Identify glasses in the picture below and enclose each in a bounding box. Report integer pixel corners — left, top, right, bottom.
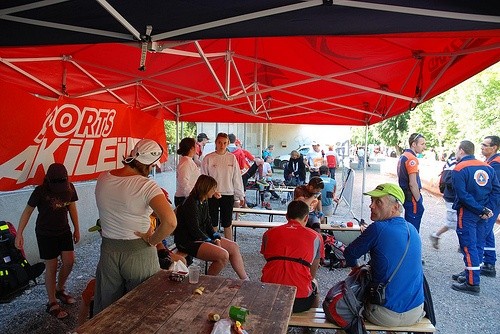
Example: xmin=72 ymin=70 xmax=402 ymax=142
xmin=481 ymin=142 xmax=490 ymax=147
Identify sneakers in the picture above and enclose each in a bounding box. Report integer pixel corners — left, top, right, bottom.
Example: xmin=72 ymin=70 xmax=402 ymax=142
xmin=256 ymin=179 xmax=269 ymax=186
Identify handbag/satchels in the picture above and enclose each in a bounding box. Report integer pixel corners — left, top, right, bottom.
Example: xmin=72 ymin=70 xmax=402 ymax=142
xmin=368 ymin=282 xmax=387 ymax=306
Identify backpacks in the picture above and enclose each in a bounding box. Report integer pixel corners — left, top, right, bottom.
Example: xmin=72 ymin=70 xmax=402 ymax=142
xmin=0 ymin=221 xmax=32 ymax=300
xmin=323 ymin=264 xmax=372 ymax=332
xmin=329 ymin=240 xmax=348 ymax=267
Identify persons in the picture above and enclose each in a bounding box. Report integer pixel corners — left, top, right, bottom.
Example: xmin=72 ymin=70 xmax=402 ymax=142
xmin=259 ymin=200 xmax=319 ymax=312
xmin=174 ymin=137 xmax=202 ymax=206
xmin=397 ymin=133 xmax=426 ymax=235
xmin=15 ymin=163 xmax=80 ymax=319
xmin=430 ymin=134 xmax=500 ymax=294
xmin=342 ymin=183 xmax=425 ymax=327
xmin=196 ymin=132 xmax=384 ymax=234
xmin=94 ymin=139 xmax=177 ymax=319
xmin=173 ymin=174 xmax=251 ymax=281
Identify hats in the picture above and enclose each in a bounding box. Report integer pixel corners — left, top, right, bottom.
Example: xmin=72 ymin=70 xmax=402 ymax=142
xmin=198 ymin=133 xmax=210 ymax=140
xmin=362 ymin=183 xmax=405 ymax=204
xmin=312 ymin=139 xmax=320 ymax=145
xmin=132 ymin=138 xmax=161 ymax=170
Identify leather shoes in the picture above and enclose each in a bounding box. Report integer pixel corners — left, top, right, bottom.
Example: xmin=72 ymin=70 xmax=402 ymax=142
xmin=451 ymin=281 xmax=480 ymax=296
xmin=458 ymin=276 xmax=466 ymax=283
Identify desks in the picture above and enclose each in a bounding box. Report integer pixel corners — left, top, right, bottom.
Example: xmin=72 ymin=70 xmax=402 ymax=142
xmin=72 ymin=268 xmax=297 ymax=334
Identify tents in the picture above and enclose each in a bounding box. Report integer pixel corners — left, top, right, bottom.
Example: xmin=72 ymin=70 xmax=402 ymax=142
xmin=0 ymin=0 xmax=500 ymax=236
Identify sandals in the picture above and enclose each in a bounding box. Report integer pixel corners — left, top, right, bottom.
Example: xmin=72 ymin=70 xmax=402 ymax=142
xmin=55 ymin=288 xmax=77 ymax=304
xmin=46 ymin=302 xmax=68 ymax=319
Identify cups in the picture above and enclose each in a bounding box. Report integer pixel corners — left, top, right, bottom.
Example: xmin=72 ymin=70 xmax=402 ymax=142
xmin=188 ymin=266 xmax=200 ymax=284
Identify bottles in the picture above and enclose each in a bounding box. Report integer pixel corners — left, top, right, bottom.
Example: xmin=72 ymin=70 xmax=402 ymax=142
xmin=208 ymin=313 xmax=220 ymax=321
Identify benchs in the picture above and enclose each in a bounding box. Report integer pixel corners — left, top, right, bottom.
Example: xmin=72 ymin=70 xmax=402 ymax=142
xmin=245 ymin=186 xmax=259 ymax=206
xmin=167 ymin=242 xmax=209 ymax=276
xmin=273 ymin=185 xmax=297 ymax=189
xmin=217 ymin=207 xmax=287 ymax=236
xmin=231 ymin=220 xmax=368 ymax=261
xmin=259 ymin=187 xmax=295 ymax=205
xmin=288 ymin=307 xmax=436 ymax=334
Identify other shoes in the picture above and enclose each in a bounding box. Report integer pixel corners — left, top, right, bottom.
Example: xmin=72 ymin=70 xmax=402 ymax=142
xmin=270 ymin=196 xmax=277 ymax=200
xmin=480 ymin=264 xmax=496 ymax=277
xmin=266 ymin=202 xmax=272 ymax=210
xmin=429 ymin=232 xmax=440 ymax=250
xmin=281 ymin=199 xmax=288 ymax=204
xmin=452 ymin=270 xmax=468 ymax=280
